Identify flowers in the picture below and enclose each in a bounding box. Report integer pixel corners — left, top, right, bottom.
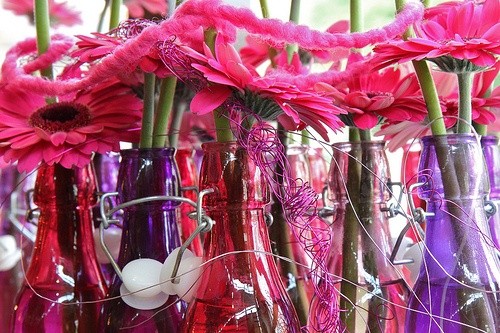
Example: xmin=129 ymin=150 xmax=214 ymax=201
xmin=0 ymin=0 xmax=500 ymax=333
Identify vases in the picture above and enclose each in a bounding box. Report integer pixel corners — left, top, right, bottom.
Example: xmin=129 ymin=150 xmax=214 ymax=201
xmin=190 ymin=135 xmax=303 ymax=333
xmin=178 ymin=148 xmax=204 ymax=255
xmin=97 ymin=144 xmax=195 ymax=333
xmin=306 ymin=139 xmax=409 ymax=333
xmin=405 ymin=130 xmax=500 ymax=333
xmin=8 ymin=152 xmax=110 ymax=333
xmin=259 ymin=140 xmax=322 ymax=321
xmin=484 ymin=132 xmax=500 ymax=248
